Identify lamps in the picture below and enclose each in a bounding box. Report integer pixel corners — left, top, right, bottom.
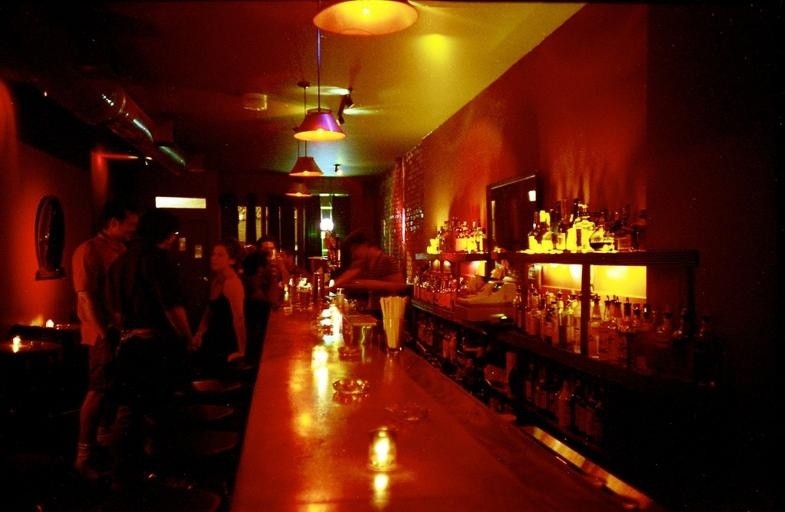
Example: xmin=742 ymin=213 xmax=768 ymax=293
xmin=283 ymin=0 xmax=421 ymax=200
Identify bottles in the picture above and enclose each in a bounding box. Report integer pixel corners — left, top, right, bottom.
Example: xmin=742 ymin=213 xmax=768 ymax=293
xmin=438 ymin=199 xmax=649 ymax=254
xmin=413 ymin=271 xmax=716 ymax=444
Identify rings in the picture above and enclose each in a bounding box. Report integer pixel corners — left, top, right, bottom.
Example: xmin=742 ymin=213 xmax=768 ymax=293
xmin=356 ymin=281 xmax=358 ymax=283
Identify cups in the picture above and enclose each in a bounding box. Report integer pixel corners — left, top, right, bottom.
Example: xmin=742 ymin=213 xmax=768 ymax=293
xmin=381 ymin=317 xmax=403 ymax=348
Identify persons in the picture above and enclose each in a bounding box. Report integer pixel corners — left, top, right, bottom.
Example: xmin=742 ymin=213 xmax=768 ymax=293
xmin=324 ymin=232 xmax=407 ymax=320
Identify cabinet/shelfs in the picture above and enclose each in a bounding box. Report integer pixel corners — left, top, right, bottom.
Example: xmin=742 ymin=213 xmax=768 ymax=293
xmin=410 ymin=248 xmax=700 ymax=461
